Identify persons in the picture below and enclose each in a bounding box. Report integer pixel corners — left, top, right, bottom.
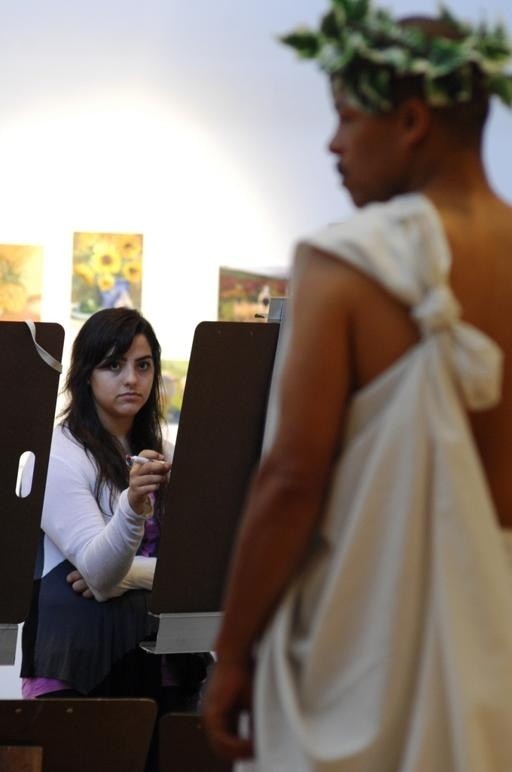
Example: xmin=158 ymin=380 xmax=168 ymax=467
xmin=199 ymin=1 xmax=511 ymax=772
xmin=13 ymin=308 xmax=220 ymax=714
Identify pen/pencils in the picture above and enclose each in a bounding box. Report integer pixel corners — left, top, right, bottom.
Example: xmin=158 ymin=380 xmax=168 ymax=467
xmin=127 ymin=456 xmax=167 ymax=464
